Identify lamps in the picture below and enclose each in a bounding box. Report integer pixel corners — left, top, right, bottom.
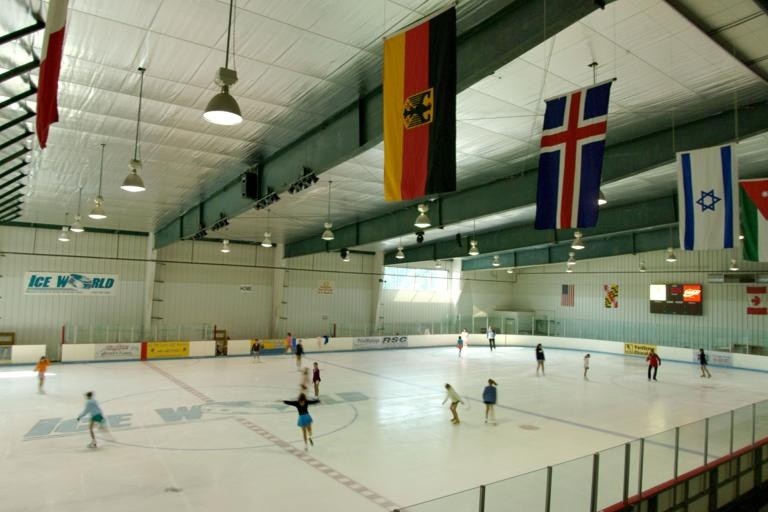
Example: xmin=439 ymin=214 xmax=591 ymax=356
xmin=219 ymin=239 xmax=231 ymax=254
xmin=57 ymin=212 xmax=72 ymax=242
xmin=87 ymin=143 xmax=109 ymax=221
xmin=339 ymin=225 xmax=746 ymax=277
xmin=118 ymin=66 xmax=147 ymax=194
xmin=321 ymin=180 xmax=334 ymax=241
xmin=260 ymin=208 xmax=273 ymax=248
xmin=198 ymin=1 xmax=245 ymax=127
xmin=70 ymin=187 xmax=85 ymax=233
xmin=413 ymin=201 xmax=433 ymax=230
xmin=586 ymin=59 xmax=608 ymax=208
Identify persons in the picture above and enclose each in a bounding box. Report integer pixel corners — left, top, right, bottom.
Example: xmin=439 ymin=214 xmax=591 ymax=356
xmin=482 ymin=378 xmax=498 ymax=424
xmin=535 ymin=344 xmax=546 ymax=376
xmin=487 ymin=326 xmax=496 ymax=350
xmin=33 ymin=356 xmax=50 ymax=391
xmin=583 ymin=353 xmax=591 ymax=380
xmin=697 ymin=347 xmax=713 ymax=378
xmin=279 ymin=332 xmax=321 ymax=450
xmin=77 ymin=392 xmax=108 ymax=449
xmin=646 ymin=348 xmax=661 ymax=382
xmin=252 ymin=339 xmax=262 ymax=361
xmin=441 ymin=383 xmax=465 ymax=426
xmin=455 ymin=335 xmax=464 ymax=359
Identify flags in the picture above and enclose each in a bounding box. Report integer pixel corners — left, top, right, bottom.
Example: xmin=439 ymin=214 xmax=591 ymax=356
xmin=561 ymin=284 xmax=575 ymax=307
xmin=532 ymin=76 xmax=617 ymax=231
xmin=382 ymin=7 xmax=458 ymax=203
xmin=738 ymin=177 xmax=768 ymax=263
xmin=675 ymin=143 xmax=741 ymax=251
xmin=746 ymin=286 xmax=768 ymax=315
xmin=35 ymin=0 xmax=69 ymax=148
